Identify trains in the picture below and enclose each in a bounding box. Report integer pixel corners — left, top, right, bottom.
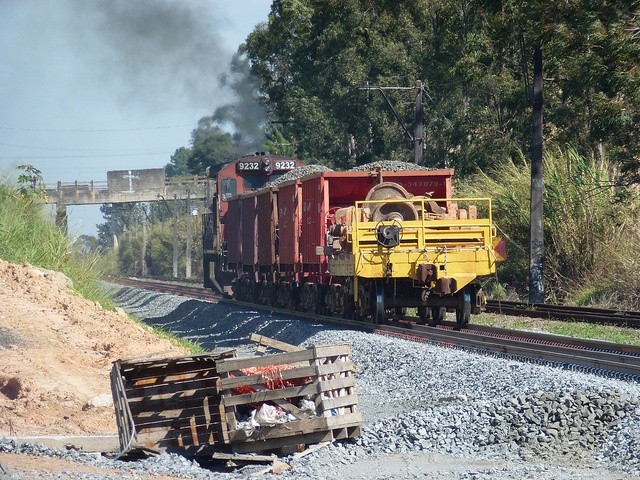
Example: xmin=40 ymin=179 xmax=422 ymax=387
xmin=202 ymin=155 xmax=497 ymax=325
